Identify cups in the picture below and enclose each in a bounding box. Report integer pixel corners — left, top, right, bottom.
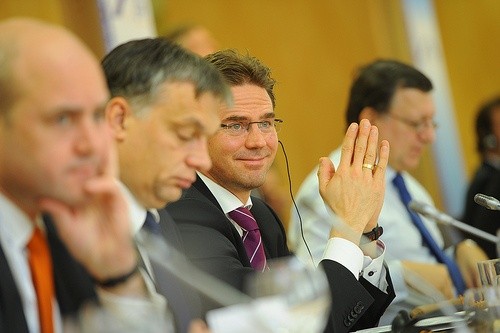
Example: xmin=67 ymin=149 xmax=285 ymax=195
xmin=475 ymin=256 xmax=500 ymax=333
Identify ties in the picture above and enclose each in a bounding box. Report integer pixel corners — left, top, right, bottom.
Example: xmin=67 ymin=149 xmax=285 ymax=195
xmin=27 ymin=227 xmax=54 ymax=333
xmin=229 ymin=208 xmax=270 ymax=273
xmin=392 ymin=172 xmax=465 ymax=296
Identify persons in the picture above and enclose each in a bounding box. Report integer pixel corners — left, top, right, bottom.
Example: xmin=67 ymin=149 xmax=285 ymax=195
xmin=0 ymin=17 xmax=500 ymax=333
xmin=162 ymin=48 xmax=397 ymax=332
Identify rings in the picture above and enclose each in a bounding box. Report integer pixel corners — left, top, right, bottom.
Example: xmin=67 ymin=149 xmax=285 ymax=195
xmin=361 ymin=163 xmax=374 ymax=172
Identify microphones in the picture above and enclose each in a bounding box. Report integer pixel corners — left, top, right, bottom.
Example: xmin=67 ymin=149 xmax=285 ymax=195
xmin=409 ymin=200 xmax=500 ymax=245
xmin=474 ymin=193 xmax=500 ymax=210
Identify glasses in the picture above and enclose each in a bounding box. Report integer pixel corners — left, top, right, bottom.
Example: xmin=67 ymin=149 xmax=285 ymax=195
xmin=386 ymin=111 xmax=439 ymax=131
xmin=220 ymin=119 xmax=283 ymax=136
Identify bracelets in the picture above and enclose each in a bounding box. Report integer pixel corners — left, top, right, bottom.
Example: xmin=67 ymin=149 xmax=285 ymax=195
xmin=359 ymin=223 xmax=383 ymax=245
